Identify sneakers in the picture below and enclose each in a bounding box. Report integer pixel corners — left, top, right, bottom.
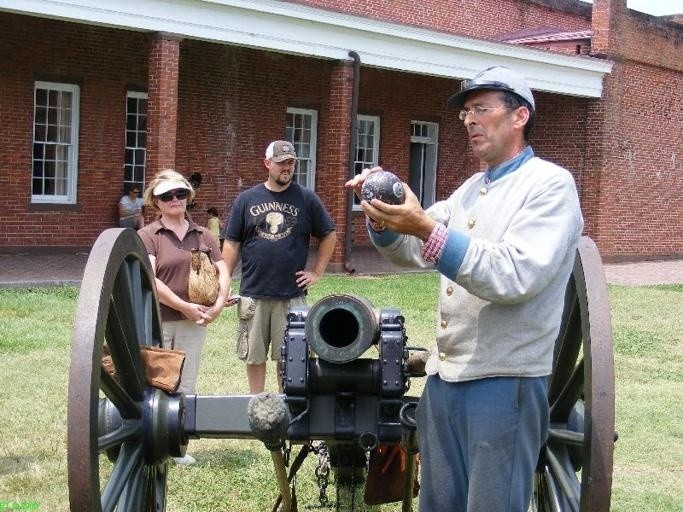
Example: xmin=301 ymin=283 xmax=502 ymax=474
xmin=173 ymin=453 xmax=196 ymax=465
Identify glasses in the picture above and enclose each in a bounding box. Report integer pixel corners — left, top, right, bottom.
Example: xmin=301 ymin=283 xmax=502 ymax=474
xmin=457 ymin=106 xmax=512 ymax=121
xmin=155 ymin=191 xmax=187 ymax=202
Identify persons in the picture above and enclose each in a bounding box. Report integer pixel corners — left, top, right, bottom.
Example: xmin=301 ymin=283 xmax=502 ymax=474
xmin=222 ymin=140 xmax=337 ymax=393
xmin=136 ymin=169 xmax=231 ymax=466
xmin=117 ymin=184 xmax=145 ymax=228
xmin=345 ymin=66 xmax=584 ymax=512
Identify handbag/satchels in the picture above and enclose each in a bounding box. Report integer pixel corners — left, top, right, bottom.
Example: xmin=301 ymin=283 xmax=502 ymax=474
xmin=186 ymin=247 xmax=220 ymax=307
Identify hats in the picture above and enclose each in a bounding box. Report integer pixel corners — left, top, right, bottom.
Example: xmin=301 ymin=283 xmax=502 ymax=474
xmin=265 ymin=140 xmax=299 ymax=165
xmin=151 ymin=179 xmax=192 ymax=196
xmin=446 ymin=66 xmax=536 ymax=114
